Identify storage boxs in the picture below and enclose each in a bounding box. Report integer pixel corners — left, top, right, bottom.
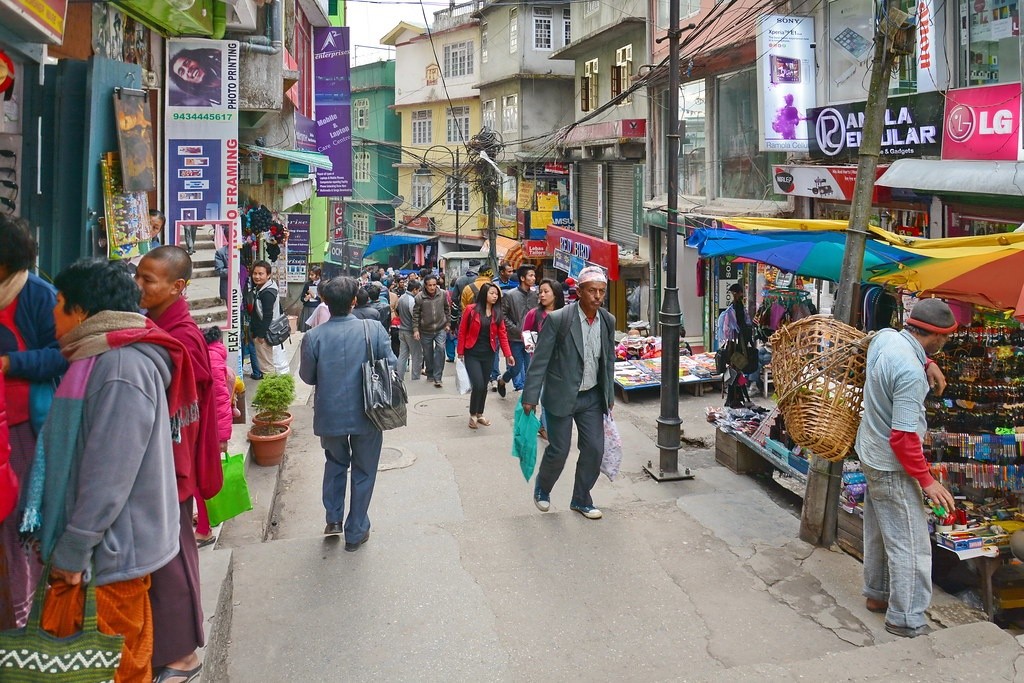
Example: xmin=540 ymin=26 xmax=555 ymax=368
xmin=715 ymin=428 xmax=776 ymax=476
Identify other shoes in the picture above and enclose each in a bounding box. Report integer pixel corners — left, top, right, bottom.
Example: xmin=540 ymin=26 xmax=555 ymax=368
xmin=885 ymin=619 xmax=931 ymax=638
xmin=492 ymin=381 xmax=497 ymax=392
xmin=865 ymin=598 xmax=888 ymax=613
xmin=423 ymin=359 xmax=453 ymax=386
xmin=250 ymin=372 xmax=260 ymax=379
xmin=345 ymin=531 xmax=369 ymax=552
xmin=324 ymin=522 xmax=343 ymax=536
xmin=498 ymin=379 xmax=506 ymax=398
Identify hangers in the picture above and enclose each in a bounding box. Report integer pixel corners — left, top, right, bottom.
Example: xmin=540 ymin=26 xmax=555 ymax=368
xmin=759 ymin=270 xmax=812 ymax=307
xmin=114 ymin=72 xmax=148 ymax=103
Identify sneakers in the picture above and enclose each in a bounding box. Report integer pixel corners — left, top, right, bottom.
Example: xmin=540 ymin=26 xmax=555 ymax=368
xmin=569 ymin=503 xmax=602 ymax=519
xmin=534 ymin=481 xmax=551 ymax=512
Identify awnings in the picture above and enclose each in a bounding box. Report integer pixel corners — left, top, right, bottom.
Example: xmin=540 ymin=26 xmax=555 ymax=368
xmin=239 ymin=143 xmax=332 ymax=172
xmin=874 ymin=159 xmax=1024 ymax=198
xmin=687 ymin=217 xmax=1024 ymax=322
xmin=480 ymin=235 xmax=523 ymax=270
xmin=363 ymin=230 xmax=438 ymax=266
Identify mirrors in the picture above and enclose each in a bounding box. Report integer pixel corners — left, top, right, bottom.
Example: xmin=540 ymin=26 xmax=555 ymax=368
xmin=175 ymin=219 xmax=234 ymax=332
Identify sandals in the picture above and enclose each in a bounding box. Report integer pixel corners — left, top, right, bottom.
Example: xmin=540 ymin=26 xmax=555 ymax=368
xmin=477 ymin=416 xmax=491 ymax=426
xmin=468 ymin=416 xmax=479 ymax=429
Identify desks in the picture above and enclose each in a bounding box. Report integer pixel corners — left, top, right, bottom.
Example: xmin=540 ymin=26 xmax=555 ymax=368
xmin=930 ymin=540 xmax=1024 ymax=622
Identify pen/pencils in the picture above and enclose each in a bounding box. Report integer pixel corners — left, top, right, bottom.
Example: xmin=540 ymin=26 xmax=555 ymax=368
xmin=924 ymin=432 xmax=1024 ymax=494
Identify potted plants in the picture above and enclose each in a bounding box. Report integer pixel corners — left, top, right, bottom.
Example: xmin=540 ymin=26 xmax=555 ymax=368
xmin=246 ymin=377 xmax=292 ymax=468
xmin=251 ymin=373 xmax=294 ymax=425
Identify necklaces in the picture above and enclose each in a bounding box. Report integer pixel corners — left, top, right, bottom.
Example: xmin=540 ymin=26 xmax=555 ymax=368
xmin=487 ymin=310 xmax=491 ymax=315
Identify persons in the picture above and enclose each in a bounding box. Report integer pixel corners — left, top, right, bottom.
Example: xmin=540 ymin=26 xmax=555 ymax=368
xmin=239 ymin=259 xmax=580 ymax=440
xmin=778 ymin=62 xmax=790 ymax=73
xmin=0 ymin=209 xmax=233 ymax=683
xmin=182 ymin=225 xmax=228 ymax=309
xmin=92 ymin=11 xmax=148 ymax=67
xmin=298 ymin=275 xmax=399 ymax=552
xmin=522 ymin=267 xmax=614 ymax=520
xmin=853 ymin=299 xmax=956 ymax=638
xmin=779 ymin=94 xmax=799 ymax=140
xmin=169 ymin=48 xmax=222 ymax=107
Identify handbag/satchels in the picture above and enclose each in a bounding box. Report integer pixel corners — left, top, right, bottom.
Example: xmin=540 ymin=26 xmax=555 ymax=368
xmin=715 ymin=330 xmax=759 ymax=388
xmin=523 ymin=330 xmax=538 ymax=353
xmin=599 ymin=408 xmax=621 ymax=483
xmin=204 ymin=450 xmax=253 ymax=528
xmin=455 ymin=356 xmax=474 ymax=396
xmin=363 ymin=318 xmax=408 ymax=432
xmin=0 ymin=557 xmax=124 ymax=683
xmin=265 ymin=313 xmax=293 ymax=350
xmin=511 ymin=393 xmax=541 ymax=483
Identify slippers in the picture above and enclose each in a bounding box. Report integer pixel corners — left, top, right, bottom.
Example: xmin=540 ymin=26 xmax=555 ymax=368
xmin=193 ymin=533 xmax=216 ymax=548
xmin=193 ymin=515 xmax=218 ymax=527
xmin=153 ymin=661 xmax=204 ymax=683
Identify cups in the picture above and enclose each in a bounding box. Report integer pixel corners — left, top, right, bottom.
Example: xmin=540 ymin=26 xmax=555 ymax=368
xmin=935 ymin=524 xmax=952 ymax=533
xmin=954 ymin=524 xmax=967 ymax=530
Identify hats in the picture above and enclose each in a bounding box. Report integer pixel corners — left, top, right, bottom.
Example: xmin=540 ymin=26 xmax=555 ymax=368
xmin=579 ymin=266 xmax=607 ymax=286
xmin=905 ymin=296 xmax=957 ymax=334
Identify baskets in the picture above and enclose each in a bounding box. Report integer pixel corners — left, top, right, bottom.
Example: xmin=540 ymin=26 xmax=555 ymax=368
xmin=765 ymin=313 xmax=874 ymax=464
